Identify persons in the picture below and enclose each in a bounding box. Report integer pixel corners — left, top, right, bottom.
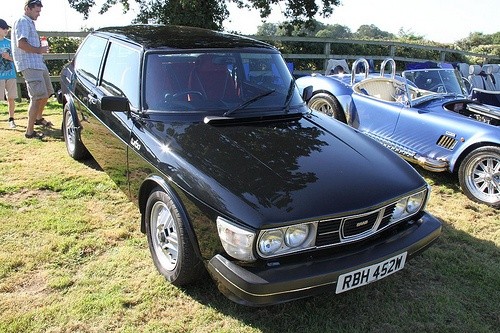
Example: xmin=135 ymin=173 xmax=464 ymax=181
xmin=11 ymin=0 xmax=54 ymax=139
xmin=0 ymin=18 xmax=17 ymax=128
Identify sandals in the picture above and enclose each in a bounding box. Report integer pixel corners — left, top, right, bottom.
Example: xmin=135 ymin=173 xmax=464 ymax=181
xmin=35 ymin=117 xmax=53 ymax=127
xmin=24 ymin=130 xmax=45 ymax=139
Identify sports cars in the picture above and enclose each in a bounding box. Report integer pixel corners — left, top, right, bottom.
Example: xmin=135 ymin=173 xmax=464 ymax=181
xmin=292 ymin=57 xmax=500 ymax=207
xmin=407 ymin=60 xmax=500 ymax=109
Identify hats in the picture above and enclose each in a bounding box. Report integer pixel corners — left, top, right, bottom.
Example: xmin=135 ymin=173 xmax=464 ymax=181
xmin=0 ymin=19 xmax=12 ymax=29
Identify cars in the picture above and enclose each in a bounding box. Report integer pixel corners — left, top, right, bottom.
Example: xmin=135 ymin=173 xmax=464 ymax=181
xmin=56 ymin=25 xmax=444 ymax=308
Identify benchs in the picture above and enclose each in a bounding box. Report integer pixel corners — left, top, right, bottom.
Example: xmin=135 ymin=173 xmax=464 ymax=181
xmin=352 ymin=77 xmax=420 ymax=100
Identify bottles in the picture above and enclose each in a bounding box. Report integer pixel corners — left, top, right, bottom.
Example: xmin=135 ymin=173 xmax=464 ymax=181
xmin=41 ymin=38 xmax=48 ymax=55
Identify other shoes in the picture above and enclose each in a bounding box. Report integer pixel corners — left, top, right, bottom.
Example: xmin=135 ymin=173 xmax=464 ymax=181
xmin=8 ymin=118 xmax=16 ymax=129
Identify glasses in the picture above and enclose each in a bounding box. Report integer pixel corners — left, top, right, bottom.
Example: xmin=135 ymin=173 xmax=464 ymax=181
xmin=4 ymin=28 xmax=10 ymax=30
xmin=30 ymin=0 xmax=41 ymax=5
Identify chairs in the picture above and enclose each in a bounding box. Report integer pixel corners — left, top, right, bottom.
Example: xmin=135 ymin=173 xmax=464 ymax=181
xmin=122 ymin=53 xmax=177 ymax=109
xmin=188 ymin=54 xmax=237 ymax=101
xmin=468 ymin=65 xmax=496 ymax=91
